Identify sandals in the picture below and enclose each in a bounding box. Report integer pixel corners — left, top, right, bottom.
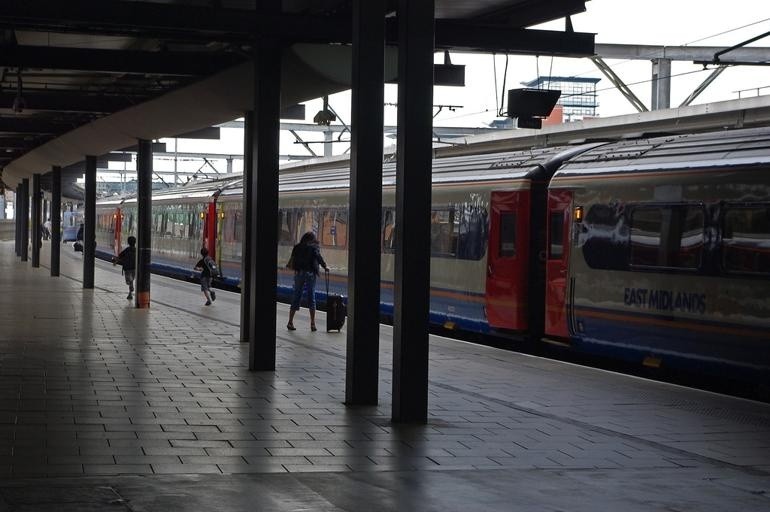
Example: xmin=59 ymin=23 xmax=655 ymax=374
xmin=286 ymin=322 xmax=297 ymax=330
xmin=309 ymin=322 xmax=317 ymax=332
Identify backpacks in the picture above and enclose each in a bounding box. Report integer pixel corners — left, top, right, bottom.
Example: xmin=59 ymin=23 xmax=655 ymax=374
xmin=203 ymin=255 xmax=219 ymax=279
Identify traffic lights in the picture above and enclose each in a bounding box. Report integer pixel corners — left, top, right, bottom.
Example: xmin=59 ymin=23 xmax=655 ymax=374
xmin=507 ymin=84 xmax=563 ymax=122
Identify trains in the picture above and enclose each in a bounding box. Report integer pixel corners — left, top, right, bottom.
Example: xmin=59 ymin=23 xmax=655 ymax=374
xmin=95 ymin=126 xmax=770 ymax=395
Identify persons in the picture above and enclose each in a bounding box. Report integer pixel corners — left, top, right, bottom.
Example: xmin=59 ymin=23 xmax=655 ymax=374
xmin=118 ymin=236 xmax=136 ymax=299
xmin=286 ymin=231 xmax=330 ymax=331
xmin=194 ymin=247 xmax=217 ymax=306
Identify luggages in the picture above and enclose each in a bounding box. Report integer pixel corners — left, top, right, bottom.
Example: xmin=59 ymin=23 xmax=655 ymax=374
xmin=325 ymin=291 xmax=347 ymax=334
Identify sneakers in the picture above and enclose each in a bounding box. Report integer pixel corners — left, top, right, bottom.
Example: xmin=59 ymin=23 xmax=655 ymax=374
xmin=129 ymin=287 xmax=134 ymax=292
xmin=210 ymin=291 xmax=216 ymax=301
xmin=126 ymin=295 xmax=133 ymax=299
xmin=205 ymin=300 xmax=211 ymax=306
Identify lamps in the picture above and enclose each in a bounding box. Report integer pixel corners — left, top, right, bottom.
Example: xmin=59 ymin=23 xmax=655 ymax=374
xmin=13 ymin=77 xmax=24 ymax=112
xmin=314 ymin=96 xmax=335 ymax=126
xmin=493 ymin=53 xmax=561 ymax=129
